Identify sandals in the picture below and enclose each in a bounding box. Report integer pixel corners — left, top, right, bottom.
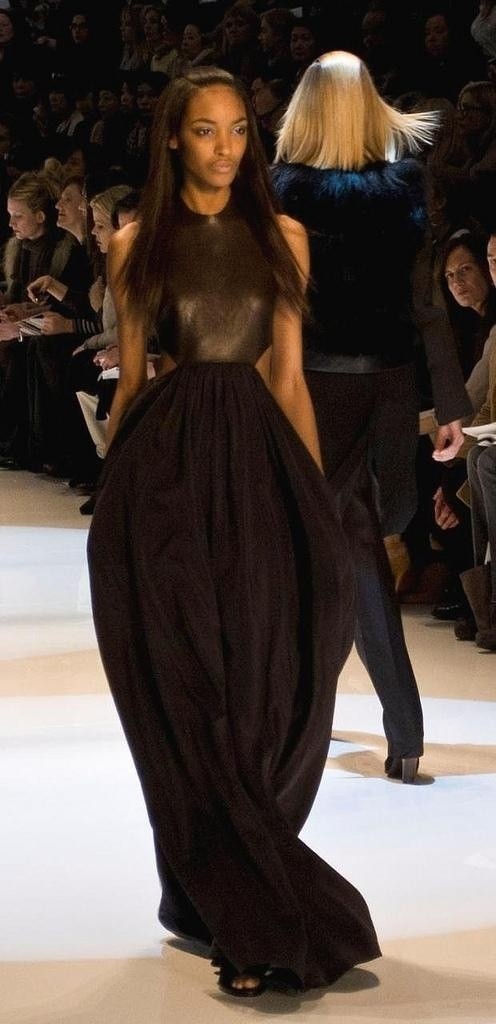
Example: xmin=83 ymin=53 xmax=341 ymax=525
xmin=218 ymin=954 xmax=268 ymax=997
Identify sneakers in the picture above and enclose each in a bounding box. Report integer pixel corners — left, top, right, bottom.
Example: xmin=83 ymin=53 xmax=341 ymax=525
xmin=476 ymin=624 xmax=495 ymax=647
xmin=453 ymin=613 xmax=476 ymax=640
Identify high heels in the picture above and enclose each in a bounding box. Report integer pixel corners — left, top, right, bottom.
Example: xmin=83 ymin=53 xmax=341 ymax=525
xmin=384 ymin=756 xmax=419 ymax=783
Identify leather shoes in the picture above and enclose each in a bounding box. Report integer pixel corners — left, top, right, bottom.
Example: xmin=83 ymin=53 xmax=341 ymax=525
xmin=430 ymin=601 xmax=464 ymax=619
xmin=79 ymin=495 xmax=96 ymax=514
